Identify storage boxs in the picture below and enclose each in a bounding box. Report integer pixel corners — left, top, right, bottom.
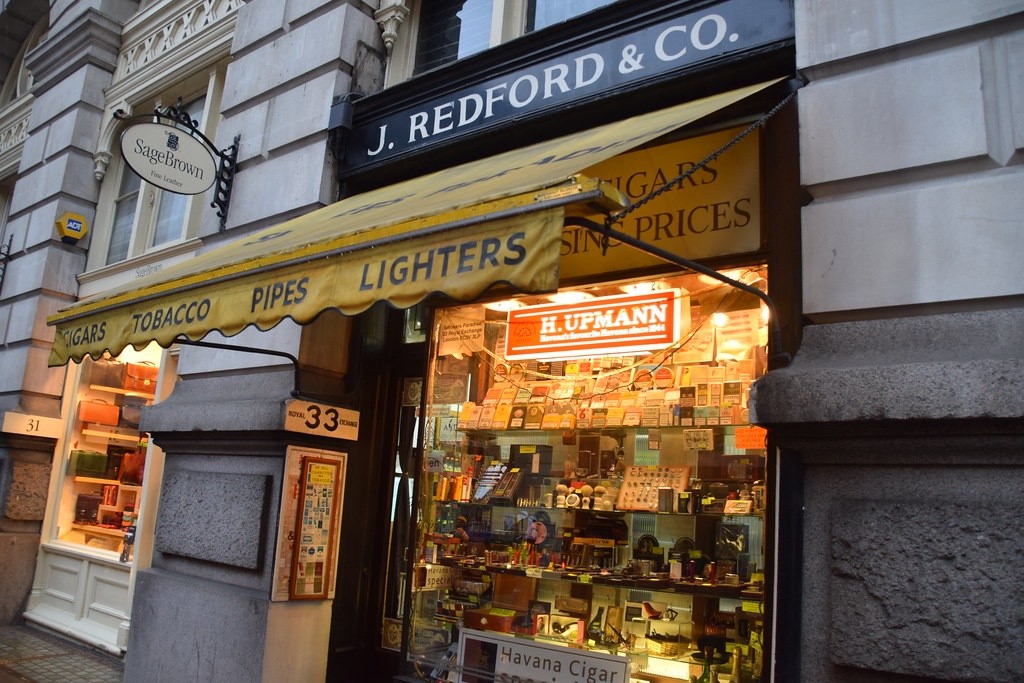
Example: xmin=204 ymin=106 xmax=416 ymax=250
xmin=463 ymin=573 xmax=540 ymax=633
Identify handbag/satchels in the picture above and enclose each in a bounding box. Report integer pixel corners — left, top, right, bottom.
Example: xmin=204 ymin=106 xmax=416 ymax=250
xmin=78 ymin=398 xmax=120 ymax=427
xmin=107 ymin=450 xmax=128 ymax=480
xmin=82 ymin=351 xmax=125 ymax=390
xmin=122 ymin=360 xmax=159 ymax=395
xmin=74 ymin=491 xmax=104 ymax=526
xmin=117 ymin=432 xmax=149 ymax=486
xmin=65 ymin=449 xmax=109 ymax=479
xmin=119 ymin=403 xmax=142 ymax=430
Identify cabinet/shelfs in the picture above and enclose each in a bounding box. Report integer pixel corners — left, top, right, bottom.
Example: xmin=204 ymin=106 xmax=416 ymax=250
xmin=425 ymin=422 xmax=772 ymax=683
xmin=70 ymin=384 xmax=156 ymax=542
